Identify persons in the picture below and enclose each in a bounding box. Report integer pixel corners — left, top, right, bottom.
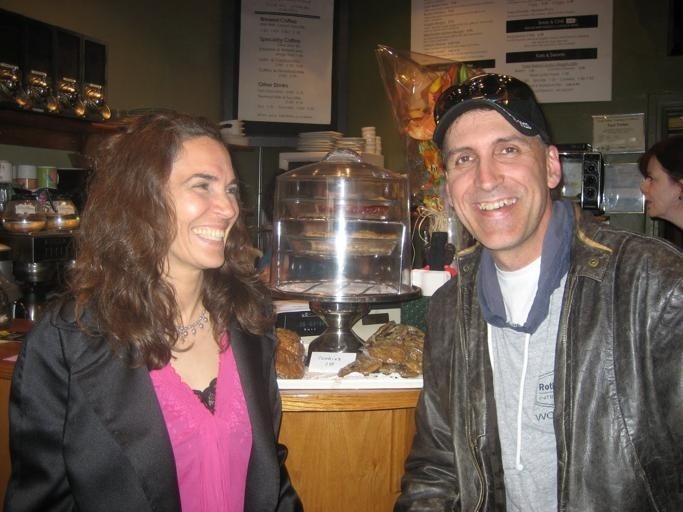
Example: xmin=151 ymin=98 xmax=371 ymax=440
xmin=393 ymin=73 xmax=683 ymax=512
xmin=638 ymin=136 xmax=683 ymax=231
xmin=4 ymin=109 xmax=304 ymax=512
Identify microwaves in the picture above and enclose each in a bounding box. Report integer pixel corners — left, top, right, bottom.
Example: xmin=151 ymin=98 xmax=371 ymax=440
xmin=553 ymin=143 xmax=604 ymax=219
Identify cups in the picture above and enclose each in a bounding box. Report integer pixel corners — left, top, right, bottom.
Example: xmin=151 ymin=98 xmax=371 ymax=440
xmin=362 ymin=127 xmax=382 ymax=154
xmin=0 ymin=160 xmax=60 ymax=210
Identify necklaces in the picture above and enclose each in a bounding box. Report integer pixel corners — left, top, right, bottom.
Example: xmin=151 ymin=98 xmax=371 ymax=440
xmin=175 ymin=309 xmax=208 ymax=336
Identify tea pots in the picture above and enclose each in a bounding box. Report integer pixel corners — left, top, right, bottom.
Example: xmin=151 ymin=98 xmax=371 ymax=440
xmin=0 ymin=186 xmax=81 ymax=234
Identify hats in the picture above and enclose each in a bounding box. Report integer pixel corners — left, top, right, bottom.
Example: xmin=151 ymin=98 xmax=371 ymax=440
xmin=432 ymin=77 xmax=551 ymax=148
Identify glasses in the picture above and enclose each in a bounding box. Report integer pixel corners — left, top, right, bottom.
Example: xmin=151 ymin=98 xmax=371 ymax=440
xmin=434 ymin=74 xmax=549 ymax=125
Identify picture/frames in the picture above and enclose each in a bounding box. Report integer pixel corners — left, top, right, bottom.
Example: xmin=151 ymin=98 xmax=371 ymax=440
xmin=232 ymin=1 xmax=339 ymax=132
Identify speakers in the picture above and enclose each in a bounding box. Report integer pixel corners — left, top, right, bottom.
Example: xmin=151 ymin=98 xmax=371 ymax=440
xmin=581 ymin=153 xmax=603 ymax=215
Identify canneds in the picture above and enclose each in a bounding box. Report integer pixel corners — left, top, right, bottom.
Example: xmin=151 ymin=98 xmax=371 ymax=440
xmin=1 ymin=194 xmax=81 ymax=233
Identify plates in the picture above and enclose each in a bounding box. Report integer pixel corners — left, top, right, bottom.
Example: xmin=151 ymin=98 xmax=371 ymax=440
xmin=218 ymin=119 xmax=247 ymax=139
xmin=294 ymin=131 xmax=366 ymax=155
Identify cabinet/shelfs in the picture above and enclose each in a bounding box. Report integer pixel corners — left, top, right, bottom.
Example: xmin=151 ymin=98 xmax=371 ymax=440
xmin=268 ymin=150 xmax=422 ymax=365
